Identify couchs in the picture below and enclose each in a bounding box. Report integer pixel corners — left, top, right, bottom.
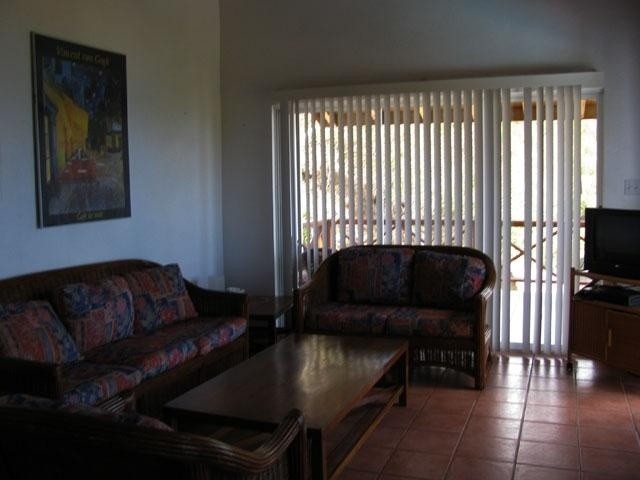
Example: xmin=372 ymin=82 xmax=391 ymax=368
xmin=2 ymin=257 xmax=250 ymax=414
xmin=1 ymin=390 xmax=310 ymax=480
xmin=294 ymin=243 xmax=494 ymax=390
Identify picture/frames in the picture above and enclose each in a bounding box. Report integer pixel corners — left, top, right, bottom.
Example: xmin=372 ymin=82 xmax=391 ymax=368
xmin=31 ymin=30 xmax=131 ymax=227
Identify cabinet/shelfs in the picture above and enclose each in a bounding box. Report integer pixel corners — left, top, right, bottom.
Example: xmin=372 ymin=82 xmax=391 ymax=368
xmin=567 ymin=270 xmax=639 ymax=375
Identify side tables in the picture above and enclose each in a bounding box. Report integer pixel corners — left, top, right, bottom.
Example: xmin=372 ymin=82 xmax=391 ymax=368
xmin=239 ymin=294 xmax=298 ymax=349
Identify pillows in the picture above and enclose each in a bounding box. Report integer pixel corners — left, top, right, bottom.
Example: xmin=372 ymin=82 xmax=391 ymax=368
xmin=5 ymin=263 xmax=198 ymax=374
xmin=335 ymin=247 xmax=481 ymax=310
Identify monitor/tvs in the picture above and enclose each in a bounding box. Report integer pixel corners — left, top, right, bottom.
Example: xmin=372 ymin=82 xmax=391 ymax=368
xmin=583 ymin=207 xmax=640 ymax=281
